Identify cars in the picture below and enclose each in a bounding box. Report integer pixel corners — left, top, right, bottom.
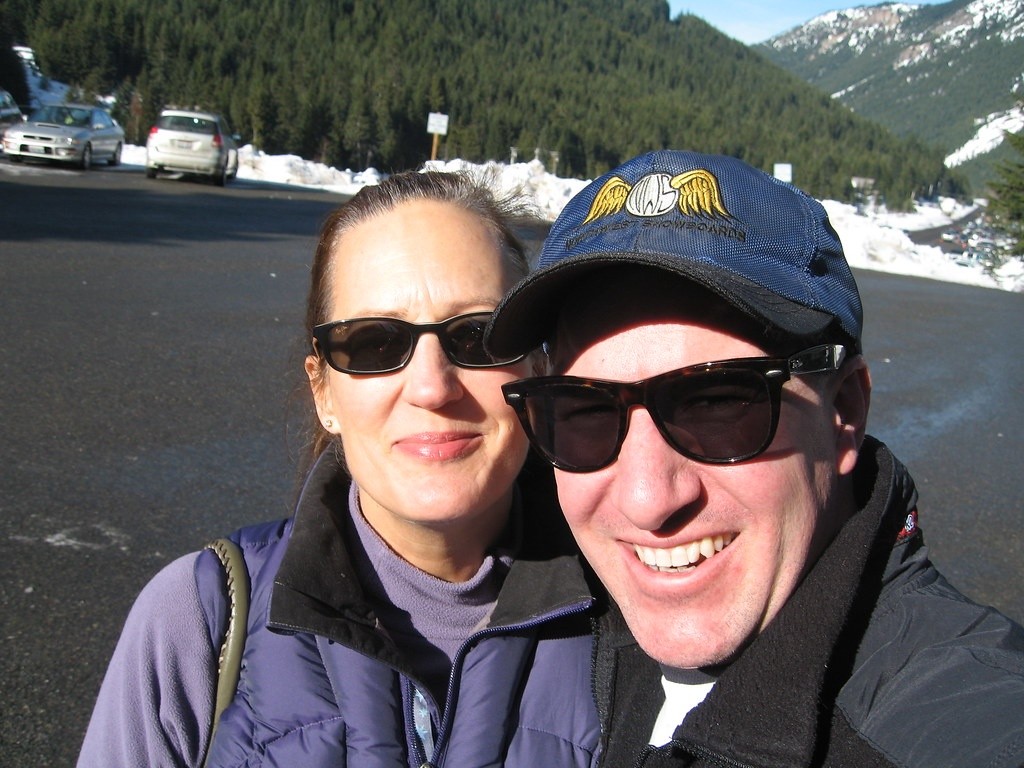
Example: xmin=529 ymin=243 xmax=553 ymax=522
xmin=143 ymin=110 xmax=242 ymax=187
xmin=0 ymin=89 xmax=125 ymax=171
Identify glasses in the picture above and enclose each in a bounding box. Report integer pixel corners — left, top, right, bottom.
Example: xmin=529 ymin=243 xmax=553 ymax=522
xmin=501 ymin=344 xmax=850 ymax=474
xmin=312 ymin=311 xmax=533 ymax=376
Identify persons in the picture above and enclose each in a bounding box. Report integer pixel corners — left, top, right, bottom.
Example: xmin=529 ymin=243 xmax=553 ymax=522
xmin=481 ymin=148 xmax=1023 ymax=767
xmin=78 ymin=164 xmax=601 ymax=767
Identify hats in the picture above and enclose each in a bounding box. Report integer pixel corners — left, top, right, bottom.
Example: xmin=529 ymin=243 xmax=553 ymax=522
xmin=485 ymin=150 xmax=863 ymax=370
